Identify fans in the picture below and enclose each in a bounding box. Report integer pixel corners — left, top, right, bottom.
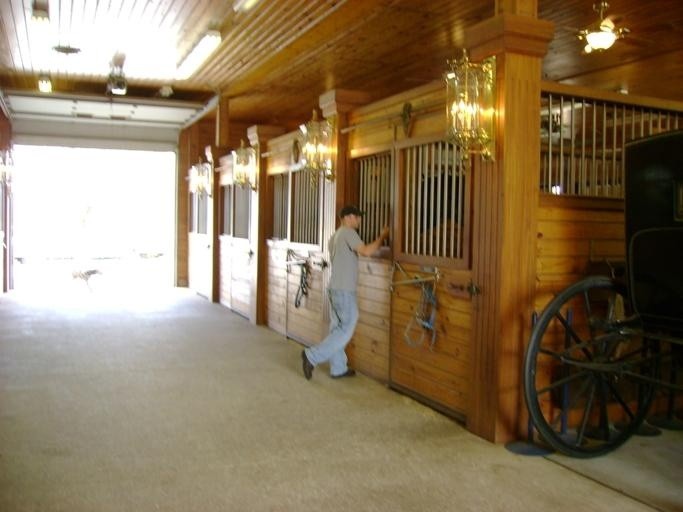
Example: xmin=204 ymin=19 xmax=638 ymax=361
xmin=564 ymin=2 xmax=654 ymax=51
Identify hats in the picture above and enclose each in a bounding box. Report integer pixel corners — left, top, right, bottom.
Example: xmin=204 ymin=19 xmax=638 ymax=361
xmin=340 ymin=206 xmax=364 ymax=218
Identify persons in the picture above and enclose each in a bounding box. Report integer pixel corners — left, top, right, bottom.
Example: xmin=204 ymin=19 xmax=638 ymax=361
xmin=300 ymin=202 xmax=388 ymax=381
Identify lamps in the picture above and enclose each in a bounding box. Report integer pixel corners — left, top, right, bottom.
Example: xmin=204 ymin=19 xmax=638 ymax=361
xmin=174 ymin=30 xmax=222 ymax=81
xmin=107 ymin=76 xmax=129 ymax=96
xmin=38 ymin=75 xmax=52 ymax=93
xmin=31 ymin=1 xmax=50 ymax=22
xmin=232 ymin=0 xmax=260 ymax=14
xmin=573 ymin=27 xmax=630 ymax=57
xmin=301 ymin=108 xmax=335 ymax=188
xmin=229 ymin=138 xmax=257 ymax=194
xmin=189 ymin=155 xmax=212 ymax=199
xmin=441 ymin=48 xmax=497 ymax=163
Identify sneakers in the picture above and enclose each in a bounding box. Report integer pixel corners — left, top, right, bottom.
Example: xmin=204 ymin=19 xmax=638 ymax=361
xmin=302 ymin=350 xmax=313 ymax=380
xmin=332 ymin=369 xmax=356 ymax=379
